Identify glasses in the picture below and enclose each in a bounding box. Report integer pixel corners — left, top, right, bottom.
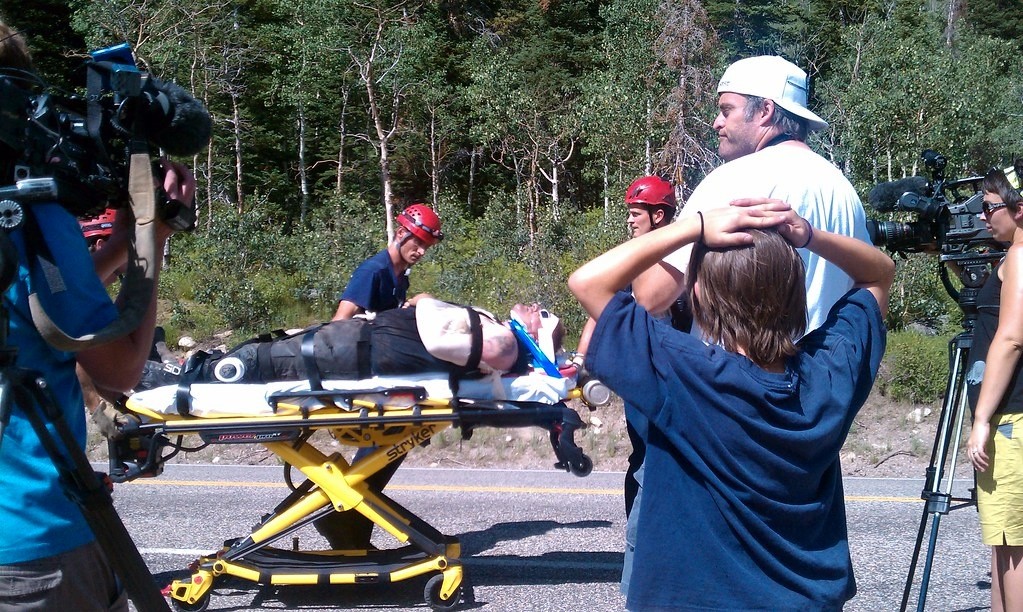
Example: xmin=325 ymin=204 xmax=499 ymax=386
xmin=539 ymin=309 xmax=549 ymax=319
xmin=982 ymin=201 xmax=1008 ymax=214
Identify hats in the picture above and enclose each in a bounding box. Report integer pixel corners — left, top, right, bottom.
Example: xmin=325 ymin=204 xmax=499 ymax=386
xmin=717 ymin=55 xmax=830 ymax=130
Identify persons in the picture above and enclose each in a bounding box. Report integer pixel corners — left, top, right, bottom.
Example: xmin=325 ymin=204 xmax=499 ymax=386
xmin=0 ymin=22 xmax=196 ymax=612
xmin=559 ymin=175 xmax=693 ymax=525
xmin=568 ymin=197 xmax=896 ymax=612
xmin=966 ymin=166 xmax=1023 ymax=612
xmin=312 ymin=204 xmax=445 ymax=551
xmin=618 ymin=54 xmax=875 ymax=599
xmin=132 ymin=292 xmax=565 ymax=393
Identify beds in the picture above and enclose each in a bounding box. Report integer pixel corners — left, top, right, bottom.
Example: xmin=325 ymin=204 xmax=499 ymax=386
xmin=108 ymin=374 xmax=610 ymax=612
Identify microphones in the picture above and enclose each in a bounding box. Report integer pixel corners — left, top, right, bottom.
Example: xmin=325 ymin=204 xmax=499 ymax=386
xmin=868 ymin=176 xmax=933 ymax=213
xmin=125 ymin=78 xmax=214 ymax=157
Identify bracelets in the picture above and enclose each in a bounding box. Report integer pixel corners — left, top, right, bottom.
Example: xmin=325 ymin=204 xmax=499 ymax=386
xmin=800 ymin=218 xmax=813 ymax=248
xmin=697 ymin=211 xmax=704 ymax=234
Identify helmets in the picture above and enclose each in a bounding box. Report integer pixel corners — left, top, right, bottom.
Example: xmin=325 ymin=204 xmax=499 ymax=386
xmin=78 ymin=208 xmax=118 ymax=239
xmin=397 ymin=204 xmax=444 ymax=245
xmin=625 ymin=177 xmax=676 ymax=208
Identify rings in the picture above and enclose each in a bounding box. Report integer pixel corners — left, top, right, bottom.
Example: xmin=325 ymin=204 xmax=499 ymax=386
xmin=972 ymin=448 xmax=978 ymax=454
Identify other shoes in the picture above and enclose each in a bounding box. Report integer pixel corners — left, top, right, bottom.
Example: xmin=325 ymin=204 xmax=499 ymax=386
xmin=312 ymin=510 xmax=381 ymax=557
xmin=148 ymin=326 xmax=181 ymax=368
